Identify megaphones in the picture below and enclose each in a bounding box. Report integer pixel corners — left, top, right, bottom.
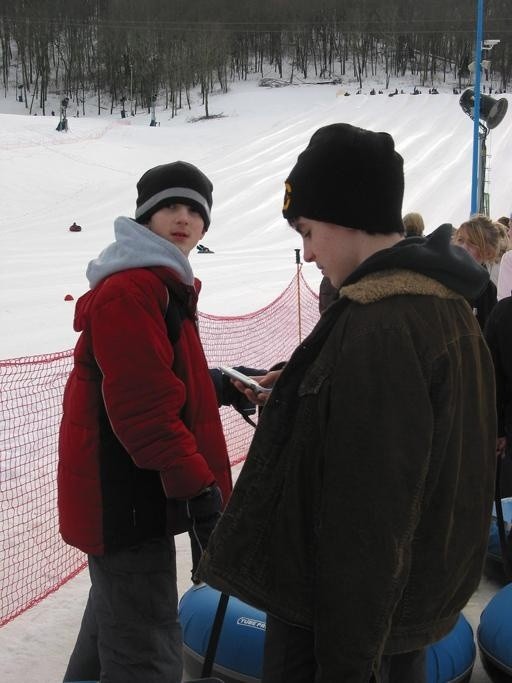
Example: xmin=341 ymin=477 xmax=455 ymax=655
xmin=459 ymin=89 xmax=508 ymax=129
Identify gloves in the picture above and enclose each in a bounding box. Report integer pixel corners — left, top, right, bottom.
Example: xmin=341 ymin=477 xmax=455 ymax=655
xmin=224 ymin=364 xmax=268 ymax=416
xmin=184 ymin=484 xmax=224 ymax=587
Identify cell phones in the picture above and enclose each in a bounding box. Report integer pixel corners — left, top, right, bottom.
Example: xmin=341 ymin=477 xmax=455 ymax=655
xmin=217 ymin=365 xmax=273 ymax=399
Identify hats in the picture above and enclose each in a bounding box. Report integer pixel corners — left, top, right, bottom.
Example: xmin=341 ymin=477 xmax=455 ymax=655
xmin=134 ymin=160 xmax=213 ymax=233
xmin=282 ymin=123 xmax=405 ymax=234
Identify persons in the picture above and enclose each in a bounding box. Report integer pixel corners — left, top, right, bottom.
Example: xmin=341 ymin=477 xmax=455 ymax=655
xmin=452 ymin=79 xmax=471 ymax=94
xmin=34 ymin=112 xmax=38 ymax=116
xmin=413 ymin=87 xmax=421 ymax=95
xmin=55 ymin=118 xmax=68 ymax=132
xmin=344 ymin=89 xmax=362 ymax=96
xmin=57 ymin=160 xmax=270 ymax=683
xmin=149 ymin=119 xmax=161 ymax=127
xmin=483 ymin=84 xmax=506 ymax=94
xmin=51 ymin=109 xmax=55 ymax=116
xmin=428 ymin=87 xmax=439 ymax=94
xmin=369 ymin=88 xmax=383 ymax=95
xmin=394 ymin=88 xmax=405 ymax=94
xmin=121 ymin=109 xmax=135 ymax=118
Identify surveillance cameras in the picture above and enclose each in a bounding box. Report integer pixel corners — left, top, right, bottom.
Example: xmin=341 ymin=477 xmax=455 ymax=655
xmin=484 ymin=39 xmax=500 ymax=46
